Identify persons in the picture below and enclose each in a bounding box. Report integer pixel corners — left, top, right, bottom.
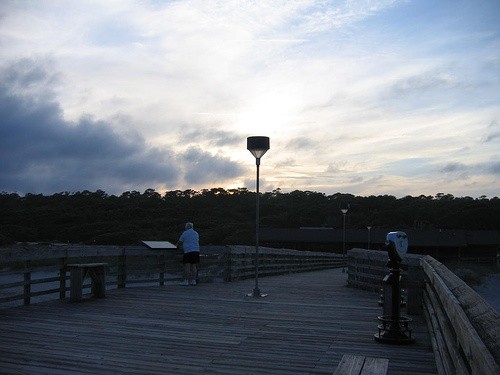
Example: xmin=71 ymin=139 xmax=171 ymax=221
xmin=177 ymin=223 xmax=199 ymax=285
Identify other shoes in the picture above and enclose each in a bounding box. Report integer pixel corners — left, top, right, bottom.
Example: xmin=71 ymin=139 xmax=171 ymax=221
xmin=179 ymin=280 xmax=196 ymax=286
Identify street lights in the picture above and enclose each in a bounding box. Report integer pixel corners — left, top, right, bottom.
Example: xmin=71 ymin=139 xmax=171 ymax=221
xmin=247 ymin=137 xmax=270 ymax=297
xmin=339 ymin=201 xmax=349 ymax=274
xmin=366 ymin=225 xmax=372 ymax=250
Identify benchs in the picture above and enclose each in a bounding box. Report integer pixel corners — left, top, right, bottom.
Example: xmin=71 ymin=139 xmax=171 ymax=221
xmin=66 ymin=262 xmax=108 ymax=302
